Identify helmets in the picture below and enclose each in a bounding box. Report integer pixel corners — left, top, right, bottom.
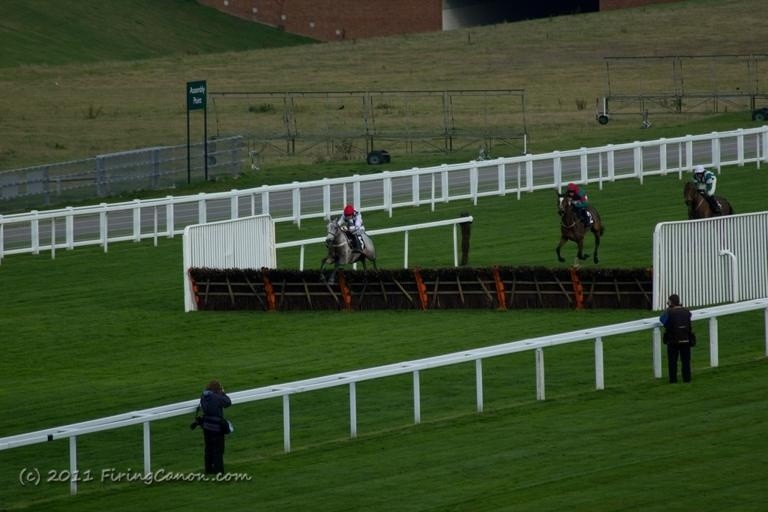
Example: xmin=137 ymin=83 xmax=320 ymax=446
xmin=694 ymin=165 xmax=705 ymax=174
xmin=344 ymin=205 xmax=355 ymax=217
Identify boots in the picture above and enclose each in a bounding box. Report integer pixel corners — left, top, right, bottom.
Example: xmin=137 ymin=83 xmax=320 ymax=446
xmin=353 ymin=239 xmax=363 ymax=253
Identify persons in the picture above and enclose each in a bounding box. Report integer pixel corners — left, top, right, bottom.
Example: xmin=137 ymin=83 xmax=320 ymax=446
xmin=659 ymin=294 xmax=693 ymax=383
xmin=200 ymin=380 xmax=231 ymax=474
xmin=694 ymin=166 xmax=718 ymax=216
xmin=337 ymin=206 xmax=365 ymax=235
xmin=568 ymin=183 xmax=588 ymax=208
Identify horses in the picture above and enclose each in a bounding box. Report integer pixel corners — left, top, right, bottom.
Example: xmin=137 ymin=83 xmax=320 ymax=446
xmin=319 ymin=214 xmax=378 ymax=285
xmin=684 ymin=181 xmax=733 ymax=220
xmin=556 ymin=190 xmax=604 ymax=264
